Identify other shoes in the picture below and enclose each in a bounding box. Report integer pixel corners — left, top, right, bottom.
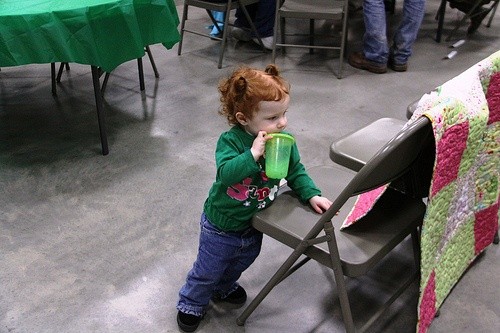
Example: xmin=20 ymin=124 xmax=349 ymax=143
xmin=176 ymin=310 xmax=201 ymax=333
xmin=222 ymin=285 xmax=247 ymax=305
xmin=232 ymin=26 xmax=255 ymax=44
xmin=467 ymin=7 xmax=490 ymax=34
xmin=349 ymin=51 xmax=388 ymax=74
xmin=388 ymin=61 xmax=409 ymax=72
xmin=252 ymin=34 xmax=282 ymax=51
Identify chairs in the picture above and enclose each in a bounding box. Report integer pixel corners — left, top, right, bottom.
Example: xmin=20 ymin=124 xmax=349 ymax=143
xmin=236 ymin=50 xmax=500 ymax=333
xmin=177 ymin=0 xmax=500 ymax=79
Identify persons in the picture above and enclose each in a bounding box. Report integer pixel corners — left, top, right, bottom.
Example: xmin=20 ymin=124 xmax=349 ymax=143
xmin=177 ymin=63 xmax=340 ymax=332
xmin=201 ymin=0 xmax=288 ymax=52
xmin=445 ymin=0 xmax=492 ymax=33
xmin=344 ymin=0 xmax=426 ymax=74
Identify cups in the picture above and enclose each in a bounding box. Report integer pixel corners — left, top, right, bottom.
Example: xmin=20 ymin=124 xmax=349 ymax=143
xmin=265 ymin=131 xmax=294 ymax=179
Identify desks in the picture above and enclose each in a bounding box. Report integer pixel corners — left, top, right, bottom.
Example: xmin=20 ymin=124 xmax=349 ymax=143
xmin=0 ymin=0 xmax=181 ymax=155
